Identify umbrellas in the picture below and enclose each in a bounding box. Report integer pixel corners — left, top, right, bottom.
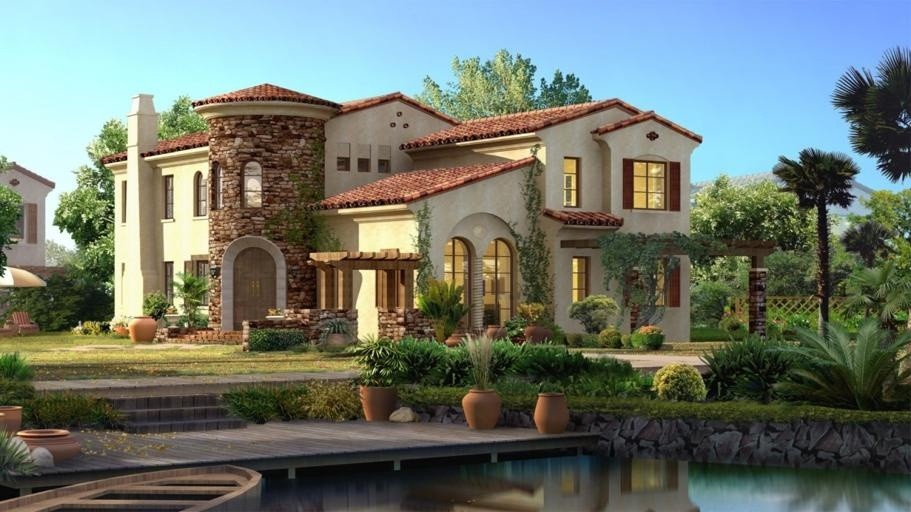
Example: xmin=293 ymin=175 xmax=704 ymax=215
xmin=0 ymin=265 xmax=48 ymax=288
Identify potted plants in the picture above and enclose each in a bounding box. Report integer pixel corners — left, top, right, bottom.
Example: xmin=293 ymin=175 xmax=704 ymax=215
xmin=461 ymin=333 xmax=502 ymax=431
xmin=354 ymin=333 xmax=407 ymax=421
xmin=316 ymin=318 xmax=355 ymax=347
xmin=519 ymin=304 xmax=551 ymax=345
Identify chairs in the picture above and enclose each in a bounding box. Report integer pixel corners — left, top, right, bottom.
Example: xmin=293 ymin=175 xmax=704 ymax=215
xmin=12 ymin=311 xmax=37 ymax=337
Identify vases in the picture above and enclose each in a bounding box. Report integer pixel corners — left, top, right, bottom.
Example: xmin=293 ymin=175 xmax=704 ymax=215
xmin=17 ymin=429 xmax=81 ymax=465
xmin=485 ymin=324 xmax=508 ymax=342
xmin=533 ymin=393 xmax=570 ymax=434
xmin=0 ymin=405 xmax=24 ymax=432
xmin=128 ymin=317 xmax=157 ymax=342
xmin=446 ymin=333 xmax=468 ymax=348
